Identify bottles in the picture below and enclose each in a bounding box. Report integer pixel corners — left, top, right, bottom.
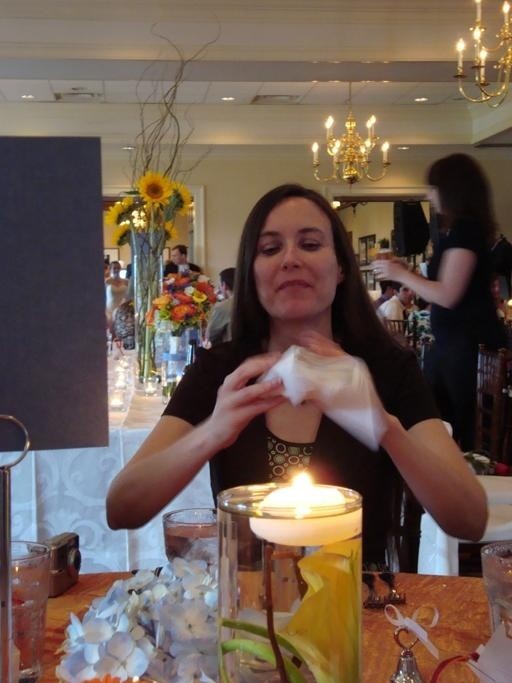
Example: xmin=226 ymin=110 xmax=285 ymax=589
xmin=163 ymin=336 xmax=198 ymax=404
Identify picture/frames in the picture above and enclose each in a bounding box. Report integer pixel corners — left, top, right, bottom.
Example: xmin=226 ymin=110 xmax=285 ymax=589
xmin=356 ymin=234 xmax=378 ymax=291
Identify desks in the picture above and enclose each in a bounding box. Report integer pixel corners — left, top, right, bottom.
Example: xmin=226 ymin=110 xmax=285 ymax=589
xmin=1 ymin=559 xmax=512 ymax=683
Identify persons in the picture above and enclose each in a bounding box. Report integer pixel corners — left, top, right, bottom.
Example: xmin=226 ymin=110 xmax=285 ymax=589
xmin=104 ymin=258 xmax=110 ymax=277
xmin=163 ymin=245 xmax=200 ymax=276
xmin=372 ymin=214 xmax=447 ymax=358
xmin=369 ymin=152 xmax=499 ymax=455
xmin=492 ymin=225 xmax=512 ymax=300
xmin=203 ymin=268 xmax=236 ymax=343
xmin=105 ymin=261 xmax=129 ymax=330
xmin=106 ymin=185 xmax=488 ymax=579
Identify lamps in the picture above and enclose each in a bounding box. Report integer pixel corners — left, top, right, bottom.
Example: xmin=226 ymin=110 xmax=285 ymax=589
xmin=308 ymin=81 xmax=391 ymax=186
xmin=454 ymin=1 xmax=512 ymax=109
xmin=331 ymin=201 xmax=367 ymax=216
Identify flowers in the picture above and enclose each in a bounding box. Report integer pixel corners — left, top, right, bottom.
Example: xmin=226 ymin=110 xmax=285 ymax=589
xmin=146 ymin=272 xmax=214 ymax=337
xmin=211 ymin=537 xmax=364 ymax=683
xmin=107 ymin=172 xmax=194 ymax=381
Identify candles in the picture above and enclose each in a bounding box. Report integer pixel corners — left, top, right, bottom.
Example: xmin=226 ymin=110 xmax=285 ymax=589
xmin=252 ymin=470 xmax=363 ymax=545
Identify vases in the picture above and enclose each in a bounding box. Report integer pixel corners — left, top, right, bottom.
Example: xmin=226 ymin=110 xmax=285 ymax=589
xmin=130 ymin=254 xmax=164 ymax=394
xmin=163 ymin=325 xmax=191 ymax=391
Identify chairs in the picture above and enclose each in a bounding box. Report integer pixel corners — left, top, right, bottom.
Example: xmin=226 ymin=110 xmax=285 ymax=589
xmin=474 ymin=344 xmax=512 ymax=464
xmin=381 ymin=317 xmax=418 ymax=360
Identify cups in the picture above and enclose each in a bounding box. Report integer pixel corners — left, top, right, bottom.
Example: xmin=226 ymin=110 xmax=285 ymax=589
xmin=214 ymin=484 xmax=363 ymax=682
xmin=163 ymin=511 xmax=241 ymax=608
xmin=480 ymin=539 xmax=512 ymax=643
xmin=107 ymin=347 xmax=141 ymax=412
xmin=373 ymin=248 xmax=392 ymax=260
xmin=9 ymin=540 xmax=48 ymax=680
